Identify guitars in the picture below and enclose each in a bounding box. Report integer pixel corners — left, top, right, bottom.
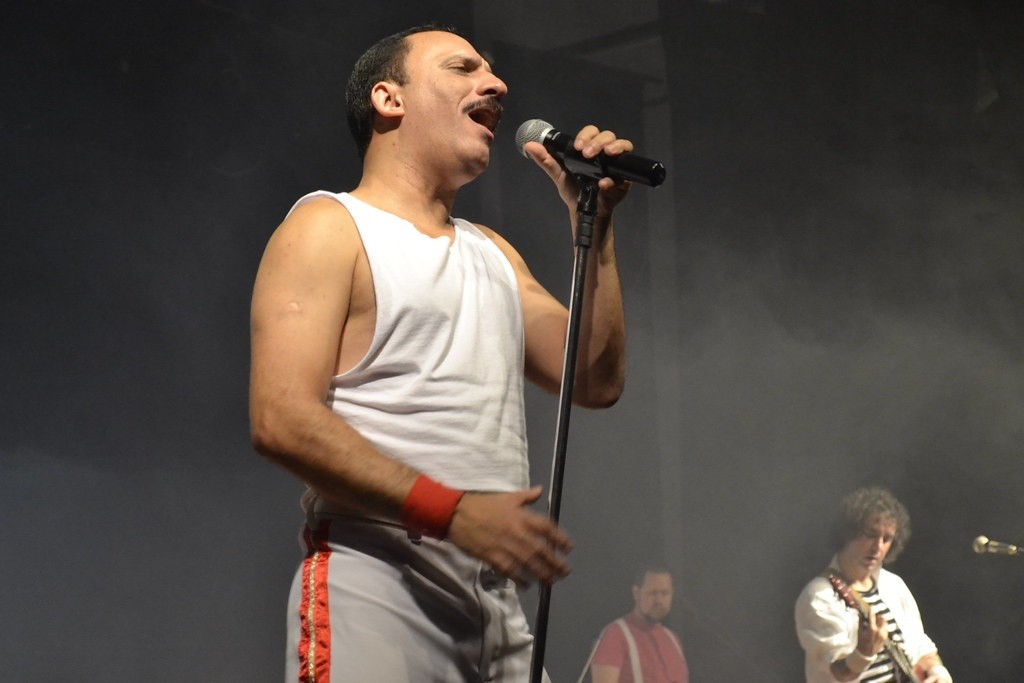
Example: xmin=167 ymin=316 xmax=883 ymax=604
xmin=827 ymin=573 xmax=928 ymax=683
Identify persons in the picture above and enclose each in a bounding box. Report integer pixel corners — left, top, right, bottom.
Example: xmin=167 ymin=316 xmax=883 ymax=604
xmin=591 ymin=566 xmax=690 ymax=683
xmin=793 ymin=486 xmax=955 ymax=683
xmin=248 ymin=24 xmax=633 ymax=683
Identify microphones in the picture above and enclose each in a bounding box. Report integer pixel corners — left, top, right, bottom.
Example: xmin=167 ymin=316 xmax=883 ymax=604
xmin=973 ymin=535 xmax=1024 ymax=555
xmin=515 ymin=118 xmax=665 ymax=188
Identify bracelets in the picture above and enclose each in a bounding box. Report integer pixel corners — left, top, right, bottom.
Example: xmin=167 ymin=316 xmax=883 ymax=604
xmin=844 ymin=647 xmax=878 ymax=674
xmin=399 ymin=473 xmax=465 ymax=541
